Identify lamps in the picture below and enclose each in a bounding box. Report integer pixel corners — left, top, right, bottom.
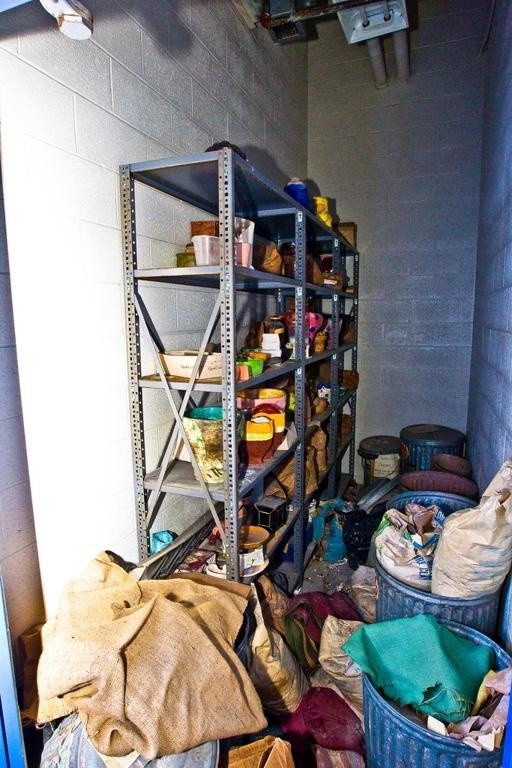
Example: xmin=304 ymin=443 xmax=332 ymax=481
xmin=332 ymin=0 xmax=410 ymax=45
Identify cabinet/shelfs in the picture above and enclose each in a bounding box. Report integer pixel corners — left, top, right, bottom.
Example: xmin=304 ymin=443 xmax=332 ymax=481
xmin=117 ymin=151 xmax=361 ymax=598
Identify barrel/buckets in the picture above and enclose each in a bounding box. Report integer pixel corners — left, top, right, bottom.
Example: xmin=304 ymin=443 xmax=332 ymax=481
xmin=385 ymin=491 xmax=477 ymax=517
xmin=401 ymin=471 xmax=477 ymax=501
xmin=374 ymin=531 xmax=499 ymax=636
xmin=400 ymin=423 xmax=465 ymax=471
xmin=361 ymin=618 xmax=512 ymax=768
xmin=357 ymin=435 xmax=401 ymax=487
xmin=182 ymin=407 xmax=225 ymax=483
